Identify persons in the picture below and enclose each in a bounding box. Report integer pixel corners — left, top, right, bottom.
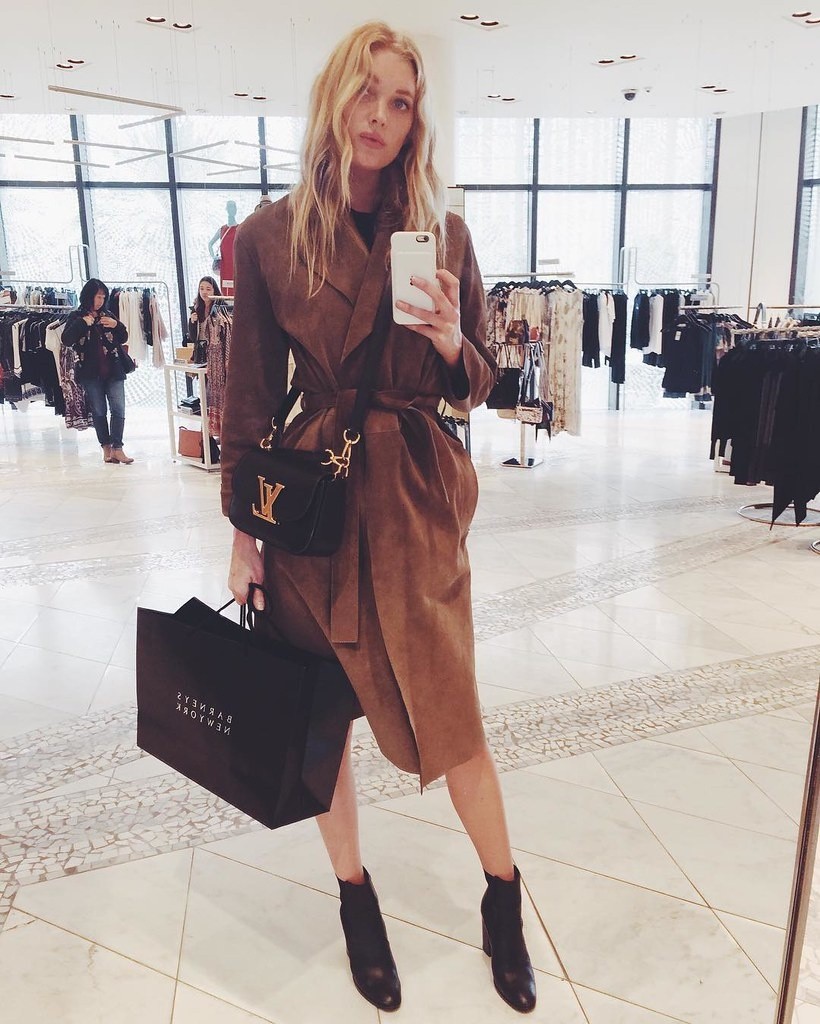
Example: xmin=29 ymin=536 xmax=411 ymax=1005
xmin=188 ymin=276 xmax=229 ymax=380
xmin=220 ymin=21 xmax=538 ymax=1013
xmin=61 ymin=279 xmax=136 ymax=464
xmin=208 ymin=200 xmax=242 ymax=296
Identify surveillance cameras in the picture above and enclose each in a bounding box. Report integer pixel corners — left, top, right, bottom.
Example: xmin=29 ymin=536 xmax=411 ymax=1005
xmin=621 ymin=89 xmax=635 ymax=101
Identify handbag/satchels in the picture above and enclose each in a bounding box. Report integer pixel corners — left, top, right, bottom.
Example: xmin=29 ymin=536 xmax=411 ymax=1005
xmin=136 ymin=582 xmax=355 ymax=830
xmin=118 ymin=342 xmax=135 ymax=374
xmin=212 ymin=256 xmax=222 ymax=273
xmin=179 ymin=429 xmax=204 ymax=457
xmin=228 ymin=447 xmax=348 ymax=558
xmin=489 ymin=341 xmax=554 ymax=428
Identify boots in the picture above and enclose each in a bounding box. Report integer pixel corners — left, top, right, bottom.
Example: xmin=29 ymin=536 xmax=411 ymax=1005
xmin=336 ymin=865 xmax=402 ymax=1011
xmin=480 ymin=864 xmax=537 ymax=1013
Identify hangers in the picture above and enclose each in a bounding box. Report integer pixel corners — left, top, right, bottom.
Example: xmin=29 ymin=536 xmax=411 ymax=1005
xmin=0 ymin=271 xmax=820 ymax=363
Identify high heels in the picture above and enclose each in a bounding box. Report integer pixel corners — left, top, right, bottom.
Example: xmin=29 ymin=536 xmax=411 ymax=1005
xmin=110 ymin=449 xmax=134 ymax=463
xmin=102 ymin=445 xmax=113 ymax=462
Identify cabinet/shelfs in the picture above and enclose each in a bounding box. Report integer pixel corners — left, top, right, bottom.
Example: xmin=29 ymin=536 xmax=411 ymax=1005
xmin=164 ymin=363 xmax=222 ymax=473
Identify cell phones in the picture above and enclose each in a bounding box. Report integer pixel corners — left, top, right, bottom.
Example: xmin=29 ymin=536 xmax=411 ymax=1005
xmin=391 ymin=231 xmax=437 ymax=325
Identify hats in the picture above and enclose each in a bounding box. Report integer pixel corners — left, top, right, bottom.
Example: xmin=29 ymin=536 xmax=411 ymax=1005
xmin=255 ymin=195 xmax=272 ymax=210
